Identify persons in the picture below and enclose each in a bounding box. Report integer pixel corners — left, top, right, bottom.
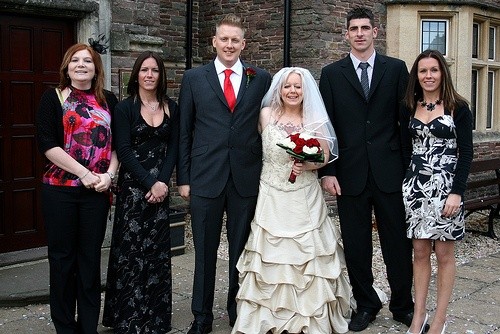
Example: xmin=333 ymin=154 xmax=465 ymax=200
xmin=35 ymin=43 xmax=120 ymax=334
xmin=175 ymin=13 xmax=272 ymax=334
xmin=319 ymin=6 xmax=430 ymax=334
xmin=230 ymin=67 xmax=358 ymax=334
xmin=399 ymin=49 xmax=473 ymax=334
xmin=102 ymin=50 xmax=180 ymax=334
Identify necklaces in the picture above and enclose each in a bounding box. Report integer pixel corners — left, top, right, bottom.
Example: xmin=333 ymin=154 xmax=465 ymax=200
xmin=419 ymin=97 xmax=441 ymax=111
xmin=149 ymin=106 xmax=158 ymax=112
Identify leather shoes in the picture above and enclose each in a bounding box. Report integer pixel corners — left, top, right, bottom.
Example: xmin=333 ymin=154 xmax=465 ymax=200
xmin=349 ymin=310 xmax=376 ymax=331
xmin=391 ymin=307 xmax=429 ymax=331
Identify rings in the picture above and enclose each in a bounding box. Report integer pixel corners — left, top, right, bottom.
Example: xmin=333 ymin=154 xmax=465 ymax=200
xmin=454 ymin=211 xmax=457 ymax=213
xmin=96 ymin=189 xmax=98 ymax=191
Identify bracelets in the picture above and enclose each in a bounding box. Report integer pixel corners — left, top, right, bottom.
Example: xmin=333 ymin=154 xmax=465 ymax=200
xmin=106 ymin=172 xmax=115 ymax=184
xmin=80 ymin=170 xmax=90 ymax=180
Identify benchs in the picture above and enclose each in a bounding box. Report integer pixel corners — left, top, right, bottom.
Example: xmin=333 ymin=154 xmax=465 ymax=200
xmin=461 ymin=157 xmax=500 ymax=241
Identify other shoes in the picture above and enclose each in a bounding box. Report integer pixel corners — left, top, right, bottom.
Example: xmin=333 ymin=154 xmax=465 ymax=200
xmin=228 ymin=318 xmax=235 ymax=327
xmin=186 ymin=322 xmax=212 ymax=334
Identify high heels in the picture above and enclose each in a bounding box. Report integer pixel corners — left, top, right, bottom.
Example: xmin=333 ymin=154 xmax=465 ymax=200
xmin=407 ymin=311 xmax=431 ymax=334
xmin=426 ymin=320 xmax=446 ymax=334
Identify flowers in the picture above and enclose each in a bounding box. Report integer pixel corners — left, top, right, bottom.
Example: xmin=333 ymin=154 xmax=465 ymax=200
xmin=277 ymin=128 xmax=325 ymax=183
xmin=244 ymin=66 xmax=257 ymax=87
xmin=88 ymin=31 xmax=112 ymax=55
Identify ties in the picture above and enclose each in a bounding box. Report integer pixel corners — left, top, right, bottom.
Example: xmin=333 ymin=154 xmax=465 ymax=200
xmin=223 ymin=70 xmax=236 ymax=112
xmin=357 ymin=62 xmax=371 ymax=100
